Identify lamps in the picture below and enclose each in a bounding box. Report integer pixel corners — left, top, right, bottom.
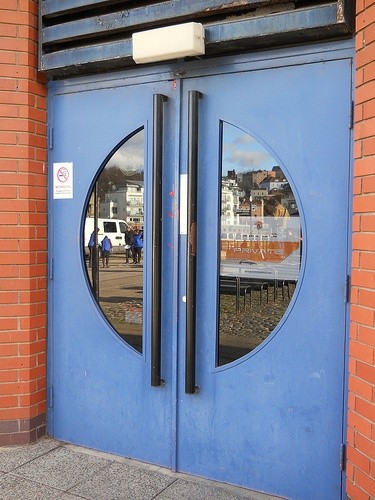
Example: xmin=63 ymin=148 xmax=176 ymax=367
xmin=132 ymin=22 xmax=205 ymax=63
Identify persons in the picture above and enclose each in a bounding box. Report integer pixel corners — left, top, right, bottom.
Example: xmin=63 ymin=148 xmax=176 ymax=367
xmin=133 ymin=231 xmax=143 ymax=263
xmin=263 ymin=199 xmax=289 ymax=256
xmin=101 ymin=236 xmax=113 ymax=268
xmin=88 ymin=228 xmax=100 ymax=266
xmin=124 ymin=226 xmax=135 ymax=263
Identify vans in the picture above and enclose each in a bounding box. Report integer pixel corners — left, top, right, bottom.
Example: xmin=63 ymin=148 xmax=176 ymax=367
xmin=84 ymin=217 xmax=133 ymax=247
